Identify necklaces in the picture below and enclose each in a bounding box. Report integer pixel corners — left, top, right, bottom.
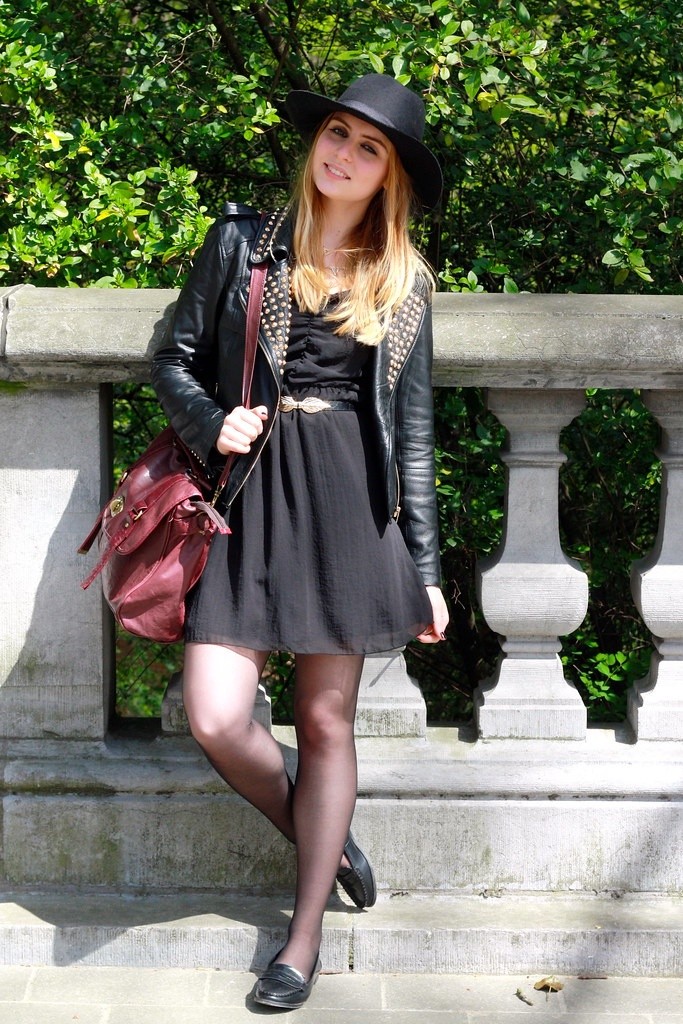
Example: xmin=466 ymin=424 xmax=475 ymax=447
xmin=322 ymin=261 xmax=348 ymax=276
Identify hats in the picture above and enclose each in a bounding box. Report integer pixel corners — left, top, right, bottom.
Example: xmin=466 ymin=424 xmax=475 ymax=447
xmin=286 ymin=74 xmax=442 ymax=214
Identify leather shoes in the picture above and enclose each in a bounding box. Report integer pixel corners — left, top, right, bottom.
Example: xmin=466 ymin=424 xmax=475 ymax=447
xmin=336 ymin=829 xmax=377 ymax=908
xmin=252 ymin=944 xmax=322 ymax=1008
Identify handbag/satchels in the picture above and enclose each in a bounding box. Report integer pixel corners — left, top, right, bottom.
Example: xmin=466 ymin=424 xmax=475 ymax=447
xmin=78 ymin=422 xmax=231 ymax=643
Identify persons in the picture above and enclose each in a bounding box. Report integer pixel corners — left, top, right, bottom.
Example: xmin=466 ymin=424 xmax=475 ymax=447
xmin=153 ymin=72 xmax=452 ymax=1010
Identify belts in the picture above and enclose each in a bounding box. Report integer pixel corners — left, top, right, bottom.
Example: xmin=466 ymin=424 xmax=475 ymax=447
xmin=279 ymin=395 xmax=358 ymax=414
